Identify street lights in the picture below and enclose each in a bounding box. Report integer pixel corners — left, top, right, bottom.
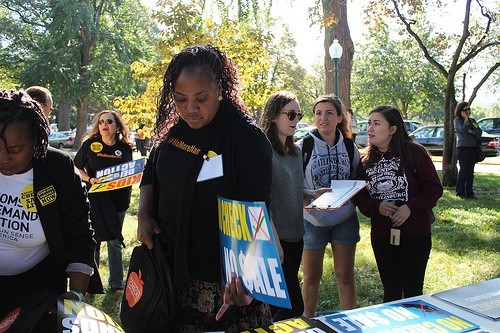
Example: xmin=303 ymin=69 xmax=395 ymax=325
xmin=329 ymin=35 xmax=343 ymax=97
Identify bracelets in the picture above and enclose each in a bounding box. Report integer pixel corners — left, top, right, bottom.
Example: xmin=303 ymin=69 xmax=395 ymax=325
xmin=88 ymin=177 xmax=91 ymax=183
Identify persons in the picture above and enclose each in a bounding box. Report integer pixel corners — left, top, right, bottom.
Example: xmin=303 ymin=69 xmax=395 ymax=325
xmin=0 ymin=89 xmax=97 ymax=333
xmin=356 ymin=105 xmax=444 ymax=304
xmin=262 ymin=90 xmax=333 ymax=321
xmin=74 ymin=110 xmax=133 ymax=299
xmin=135 ymin=118 xmax=151 ymax=156
xmin=346 ymin=108 xmax=358 ymax=142
xmin=24 ymin=85 xmax=54 ymax=117
xmin=121 ymin=44 xmax=274 ymax=333
xmin=295 ymin=93 xmax=361 ymax=319
xmin=454 ymin=102 xmax=485 ymax=200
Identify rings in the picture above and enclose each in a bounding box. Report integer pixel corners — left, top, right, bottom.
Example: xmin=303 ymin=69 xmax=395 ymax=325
xmin=386 ymin=211 xmax=389 ymax=215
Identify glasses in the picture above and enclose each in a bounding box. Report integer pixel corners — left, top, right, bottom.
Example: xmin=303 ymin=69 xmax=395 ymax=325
xmin=99 ymin=118 xmax=113 ymax=125
xmin=278 ymin=110 xmax=304 ymax=121
xmin=462 ymin=108 xmax=470 ymax=112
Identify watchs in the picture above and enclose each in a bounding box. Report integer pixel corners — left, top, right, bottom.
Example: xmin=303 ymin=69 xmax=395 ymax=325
xmin=71 ymin=287 xmax=84 ymax=301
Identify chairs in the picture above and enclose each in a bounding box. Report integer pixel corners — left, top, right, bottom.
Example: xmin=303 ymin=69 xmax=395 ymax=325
xmin=438 ymin=131 xmax=444 ymax=137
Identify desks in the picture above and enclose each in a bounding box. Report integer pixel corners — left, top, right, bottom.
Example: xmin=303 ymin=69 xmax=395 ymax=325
xmin=239 ymin=277 xmax=500 ymax=333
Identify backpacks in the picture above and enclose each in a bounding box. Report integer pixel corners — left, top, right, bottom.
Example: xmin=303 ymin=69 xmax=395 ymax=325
xmin=0 ymin=274 xmax=65 ymax=333
xmin=120 ymin=230 xmax=177 ymax=333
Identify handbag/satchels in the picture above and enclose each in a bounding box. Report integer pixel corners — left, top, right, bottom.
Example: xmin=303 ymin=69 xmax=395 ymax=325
xmin=475 ymin=145 xmax=485 ymax=162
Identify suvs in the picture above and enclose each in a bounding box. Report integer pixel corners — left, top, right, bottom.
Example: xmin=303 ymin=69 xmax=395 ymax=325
xmin=355 ymin=121 xmax=371 ymax=148
xmin=293 ymin=122 xmax=316 ymax=143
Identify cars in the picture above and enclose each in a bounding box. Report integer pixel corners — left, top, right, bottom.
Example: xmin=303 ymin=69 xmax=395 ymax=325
xmin=402 ymin=118 xmax=500 ymax=162
xmin=47 ymin=123 xmax=139 ymax=151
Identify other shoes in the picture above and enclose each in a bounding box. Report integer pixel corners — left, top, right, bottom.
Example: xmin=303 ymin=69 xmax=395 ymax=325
xmin=111 ymin=287 xmax=124 ymax=294
